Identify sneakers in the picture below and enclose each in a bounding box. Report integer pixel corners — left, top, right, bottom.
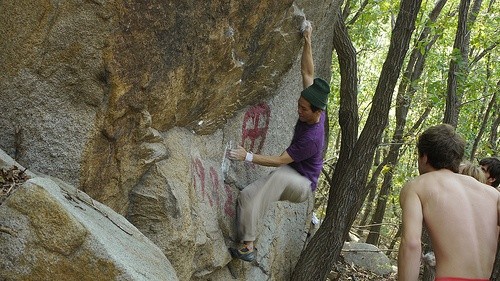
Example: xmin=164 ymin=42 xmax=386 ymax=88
xmin=228 ymin=247 xmax=255 ymax=262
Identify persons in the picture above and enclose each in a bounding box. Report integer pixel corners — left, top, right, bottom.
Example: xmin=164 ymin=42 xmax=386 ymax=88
xmin=227 ymin=26 xmax=330 ymax=262
xmin=397 ymin=124 xmax=500 ymax=281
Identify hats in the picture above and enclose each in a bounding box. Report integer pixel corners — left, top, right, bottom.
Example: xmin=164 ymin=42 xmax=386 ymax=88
xmin=301 ymin=77 xmax=331 ymax=111
